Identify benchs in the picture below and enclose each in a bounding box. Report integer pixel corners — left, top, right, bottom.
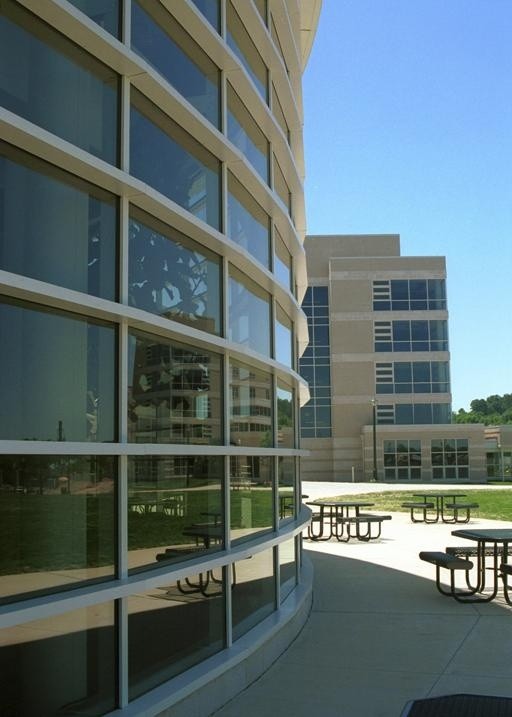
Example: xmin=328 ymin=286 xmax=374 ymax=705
xmin=155 ymin=545 xmax=253 ymax=598
xmin=302 ymin=502 xmax=512 ymax=606
xmin=284 ymin=506 xmax=293 ymax=509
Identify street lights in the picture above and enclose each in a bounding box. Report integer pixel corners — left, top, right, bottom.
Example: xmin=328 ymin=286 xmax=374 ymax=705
xmin=369 ymin=396 xmax=378 ymax=479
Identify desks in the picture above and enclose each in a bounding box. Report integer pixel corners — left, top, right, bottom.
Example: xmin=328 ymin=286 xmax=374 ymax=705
xmin=180 ymin=508 xmax=226 ymax=550
xmin=302 ymin=495 xmax=309 ymax=498
xmin=413 ymin=492 xmax=468 ymax=525
xmin=400 ymin=693 xmax=511 ymax=717
xmin=279 ymin=495 xmax=293 ymax=519
